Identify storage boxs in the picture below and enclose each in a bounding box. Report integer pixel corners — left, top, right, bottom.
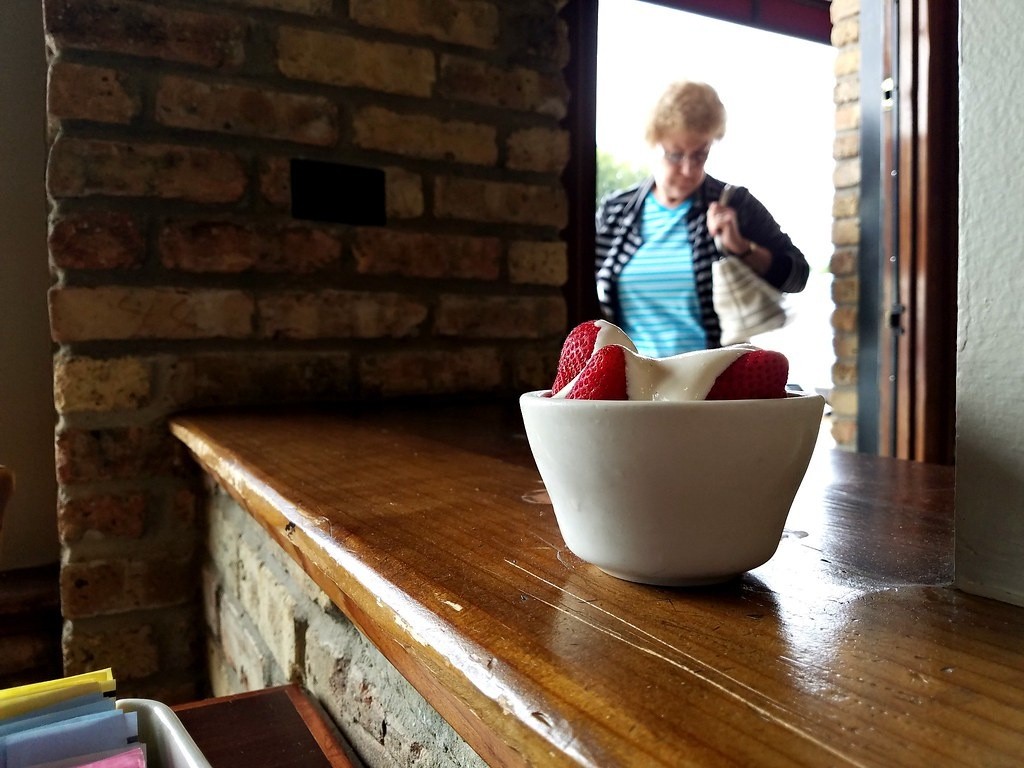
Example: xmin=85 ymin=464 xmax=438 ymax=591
xmin=0 ymin=697 xmax=212 ymax=768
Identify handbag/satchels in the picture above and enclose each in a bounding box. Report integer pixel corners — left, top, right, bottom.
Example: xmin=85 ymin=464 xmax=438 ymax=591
xmin=712 ymin=184 xmax=793 ymax=344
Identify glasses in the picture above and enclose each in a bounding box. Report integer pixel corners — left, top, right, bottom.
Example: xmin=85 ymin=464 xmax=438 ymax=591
xmin=657 ymin=142 xmax=710 ymax=167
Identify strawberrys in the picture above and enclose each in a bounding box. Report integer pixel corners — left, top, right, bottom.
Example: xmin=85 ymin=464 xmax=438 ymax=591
xmin=549 ymin=319 xmax=795 ymax=402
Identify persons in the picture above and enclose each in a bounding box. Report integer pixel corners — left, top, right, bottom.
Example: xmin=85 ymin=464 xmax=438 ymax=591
xmin=594 ymin=79 xmax=810 ymax=361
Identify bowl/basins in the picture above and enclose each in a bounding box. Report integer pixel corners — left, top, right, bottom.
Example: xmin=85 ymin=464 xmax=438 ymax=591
xmin=520 ymin=386 xmax=827 ymax=585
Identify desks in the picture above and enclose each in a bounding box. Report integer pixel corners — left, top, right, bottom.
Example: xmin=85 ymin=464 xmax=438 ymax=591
xmin=169 ymin=683 xmax=365 ymax=768
